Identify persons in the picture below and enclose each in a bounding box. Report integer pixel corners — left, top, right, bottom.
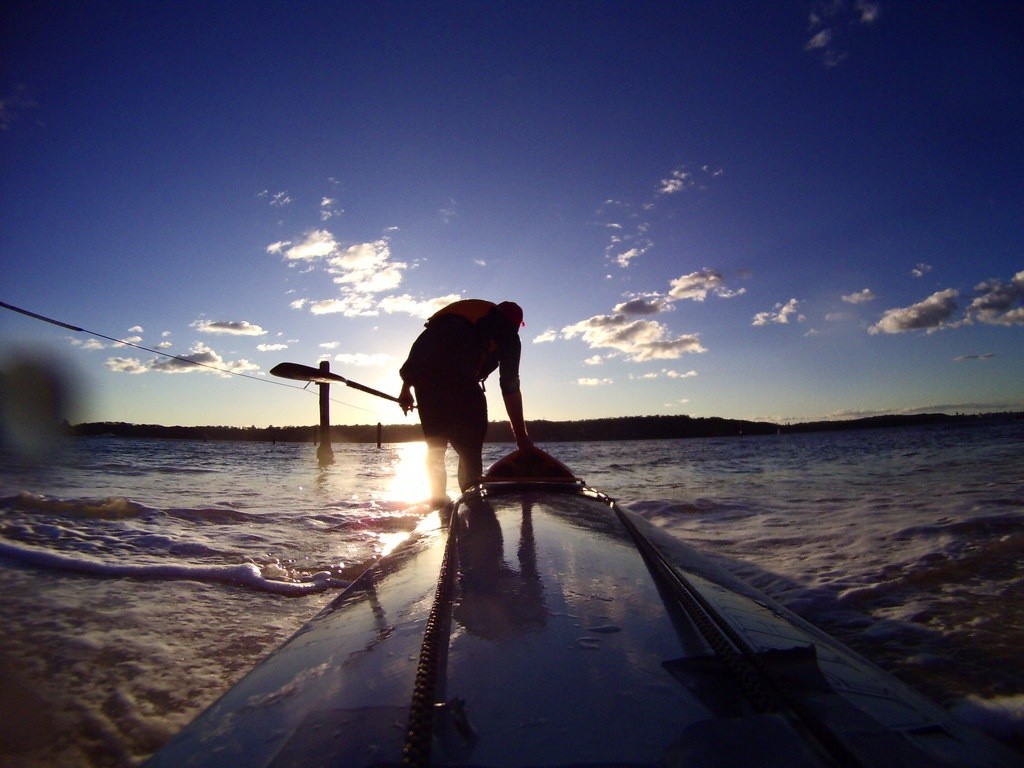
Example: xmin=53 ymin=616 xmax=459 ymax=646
xmin=396 ymin=298 xmax=530 ymax=509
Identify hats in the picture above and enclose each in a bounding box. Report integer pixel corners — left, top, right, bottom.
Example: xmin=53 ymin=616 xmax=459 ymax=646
xmin=498 ymin=301 xmax=523 ymax=332
